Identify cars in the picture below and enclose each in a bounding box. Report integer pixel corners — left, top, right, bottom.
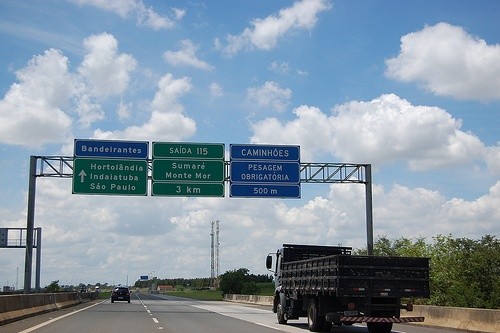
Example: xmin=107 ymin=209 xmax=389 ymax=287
xmin=111 ymin=287 xmax=131 ymax=303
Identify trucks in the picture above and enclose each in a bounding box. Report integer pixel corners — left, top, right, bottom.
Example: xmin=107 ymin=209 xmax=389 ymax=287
xmin=80 ymin=286 xmax=86 ymax=293
xmin=265 ymin=244 xmax=430 ymax=332
xmin=95 ymin=286 xmax=102 ymax=294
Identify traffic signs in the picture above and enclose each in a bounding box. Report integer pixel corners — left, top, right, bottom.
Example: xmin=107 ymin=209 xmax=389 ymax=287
xmin=73 ymin=157 xmax=149 ymax=197
